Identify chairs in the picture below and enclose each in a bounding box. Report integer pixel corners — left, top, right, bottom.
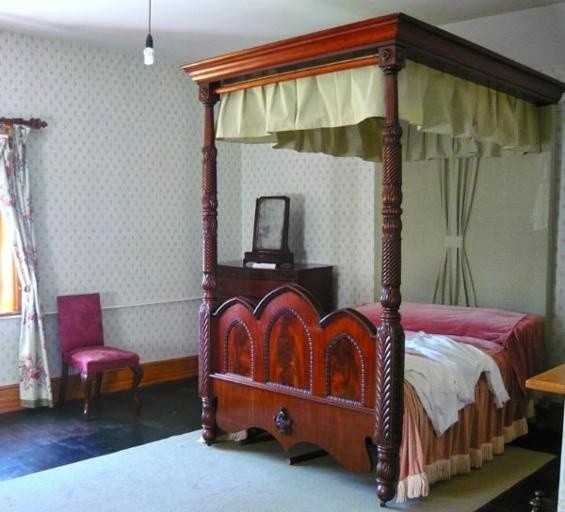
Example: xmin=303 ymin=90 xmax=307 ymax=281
xmin=56 ymin=293 xmax=143 ymax=422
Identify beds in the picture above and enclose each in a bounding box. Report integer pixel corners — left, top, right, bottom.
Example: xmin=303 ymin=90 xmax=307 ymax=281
xmin=177 ymin=10 xmax=565 ymax=510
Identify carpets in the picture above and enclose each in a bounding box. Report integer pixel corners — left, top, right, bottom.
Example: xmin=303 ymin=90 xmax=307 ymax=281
xmin=0 ymin=431 xmax=555 ymax=510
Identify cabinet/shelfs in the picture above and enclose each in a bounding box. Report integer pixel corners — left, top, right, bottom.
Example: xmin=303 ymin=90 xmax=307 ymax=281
xmin=212 ymin=258 xmax=336 ymax=338
